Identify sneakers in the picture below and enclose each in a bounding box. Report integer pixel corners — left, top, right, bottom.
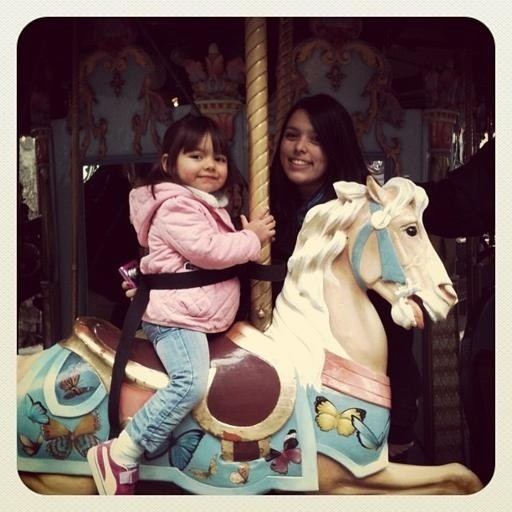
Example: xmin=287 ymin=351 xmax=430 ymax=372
xmin=87 ymin=438 xmax=138 ymax=495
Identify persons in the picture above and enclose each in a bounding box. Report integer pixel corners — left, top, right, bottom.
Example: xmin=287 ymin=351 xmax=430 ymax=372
xmin=87 ymin=113 xmax=275 ymax=495
xmin=18 ymin=182 xmax=43 ymax=303
xmin=121 ymin=94 xmax=422 ymax=464
xmin=223 ymin=161 xmax=244 ymax=230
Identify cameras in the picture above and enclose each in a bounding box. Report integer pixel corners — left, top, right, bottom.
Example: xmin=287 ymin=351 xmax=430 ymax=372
xmin=117 ymin=260 xmax=140 ymax=291
